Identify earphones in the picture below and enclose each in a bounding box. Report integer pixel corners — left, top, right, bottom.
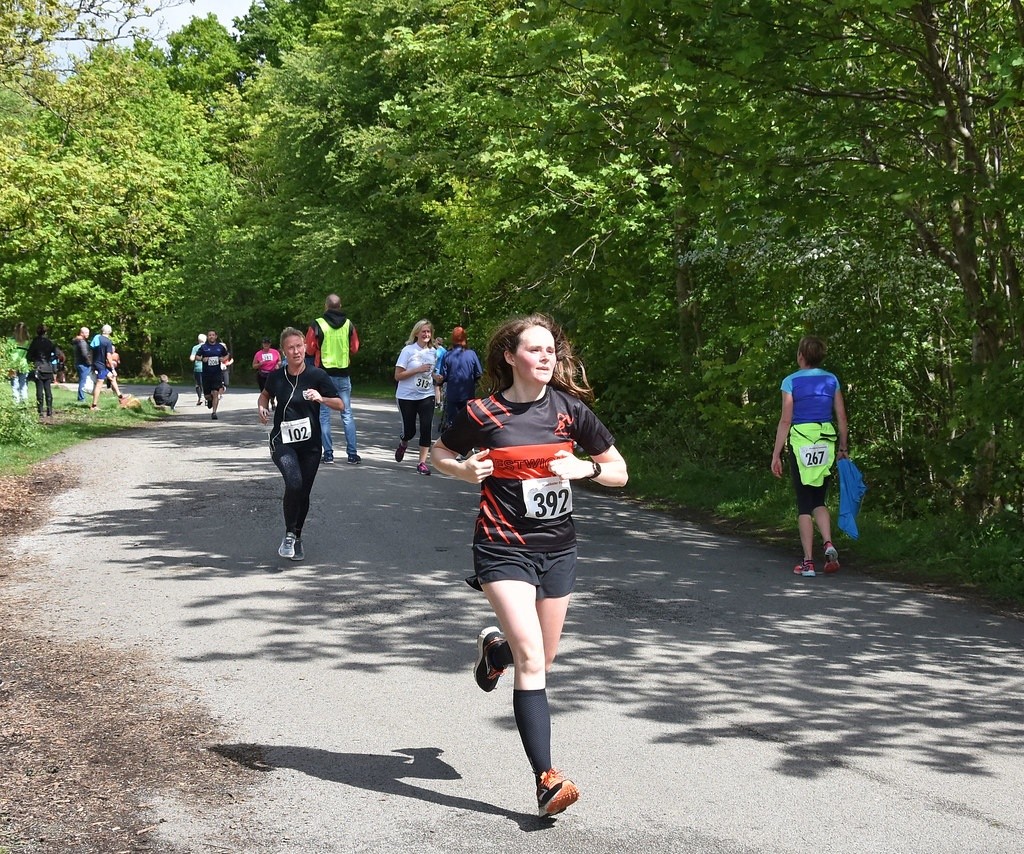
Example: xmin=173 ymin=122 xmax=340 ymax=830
xmin=304 ymin=347 xmax=305 ymax=353
xmin=283 ymin=351 xmax=285 ymax=356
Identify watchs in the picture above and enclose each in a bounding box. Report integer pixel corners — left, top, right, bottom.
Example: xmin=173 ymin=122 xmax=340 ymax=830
xmin=587 ymin=461 xmax=602 ymax=483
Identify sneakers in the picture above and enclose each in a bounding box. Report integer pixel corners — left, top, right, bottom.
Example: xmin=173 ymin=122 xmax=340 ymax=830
xmin=347 ymin=454 xmax=361 ymax=464
xmin=90 ymin=406 xmax=101 ymax=411
xmin=290 ymin=538 xmax=305 ymax=561
xmin=323 ymin=449 xmax=334 ymax=464
xmin=211 ymin=413 xmax=218 ymax=420
xmin=395 ymin=440 xmax=408 ymax=463
xmin=208 ymin=400 xmax=213 ymax=409
xmin=794 ymin=556 xmax=815 ymax=577
xmin=536 ymin=767 xmax=580 ymax=818
xmin=119 ymin=393 xmax=132 ymax=404
xmin=823 ymin=541 xmax=840 ymax=573
xmin=278 ymin=532 xmax=296 ymax=557
xmin=417 ymin=462 xmax=432 ymax=475
xmin=473 ymin=626 xmax=508 ymax=692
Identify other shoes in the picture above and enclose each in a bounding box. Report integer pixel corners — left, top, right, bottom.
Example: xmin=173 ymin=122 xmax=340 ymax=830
xmin=39 ymin=413 xmax=54 ymax=418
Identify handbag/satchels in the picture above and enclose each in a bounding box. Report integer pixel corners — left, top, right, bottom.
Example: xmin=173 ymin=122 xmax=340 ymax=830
xmin=82 ymin=371 xmax=97 ymax=394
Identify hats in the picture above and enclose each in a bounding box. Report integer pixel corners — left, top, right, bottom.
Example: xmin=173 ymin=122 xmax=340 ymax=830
xmin=262 ymin=337 xmax=272 ymax=344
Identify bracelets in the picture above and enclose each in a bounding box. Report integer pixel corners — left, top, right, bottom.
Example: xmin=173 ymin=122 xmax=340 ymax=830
xmin=839 ymin=447 xmax=848 ymax=453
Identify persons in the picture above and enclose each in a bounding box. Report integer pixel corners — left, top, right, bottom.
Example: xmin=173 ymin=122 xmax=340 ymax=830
xmin=429 ymin=310 xmax=629 ymax=821
xmin=153 ymin=374 xmax=179 ymax=413
xmin=770 ymin=334 xmax=848 ymax=577
xmin=90 ymin=324 xmax=132 ymax=411
xmin=252 ymin=336 xmax=315 ymax=413
xmin=50 ymin=344 xmax=79 ymax=385
xmin=189 ymin=329 xmax=235 ymax=420
xmin=25 ymin=323 xmax=58 ymax=420
xmin=304 ymin=293 xmax=362 ymax=465
xmin=71 ymin=327 xmax=92 ymax=403
xmin=394 ymin=317 xmax=483 ymax=475
xmin=106 ymin=345 xmax=121 ymax=391
xmin=6 ymin=322 xmax=33 ymax=410
xmin=257 ymin=327 xmax=346 ymax=561
